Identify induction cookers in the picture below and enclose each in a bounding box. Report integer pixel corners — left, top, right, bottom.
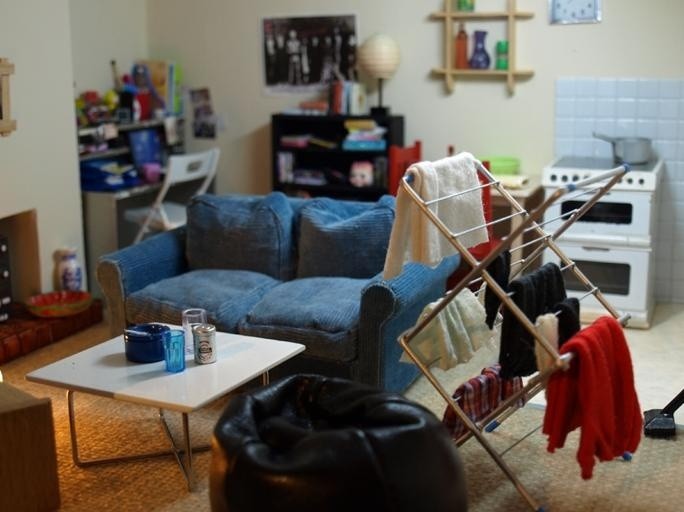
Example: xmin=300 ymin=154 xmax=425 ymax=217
xmin=542 ymin=152 xmax=663 ymax=190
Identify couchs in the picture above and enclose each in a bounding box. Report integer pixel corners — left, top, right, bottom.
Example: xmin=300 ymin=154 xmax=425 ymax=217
xmin=96 ymin=195 xmax=462 ymax=397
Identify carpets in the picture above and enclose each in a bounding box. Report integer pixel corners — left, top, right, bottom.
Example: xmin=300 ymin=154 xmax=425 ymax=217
xmin=0 ymin=297 xmax=684 ymax=512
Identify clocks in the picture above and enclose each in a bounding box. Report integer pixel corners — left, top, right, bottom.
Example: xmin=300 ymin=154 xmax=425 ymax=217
xmin=548 ymin=0 xmax=602 ymax=24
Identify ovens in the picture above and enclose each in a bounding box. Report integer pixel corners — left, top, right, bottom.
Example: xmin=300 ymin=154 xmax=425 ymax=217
xmin=544 ymin=189 xmax=665 ymax=329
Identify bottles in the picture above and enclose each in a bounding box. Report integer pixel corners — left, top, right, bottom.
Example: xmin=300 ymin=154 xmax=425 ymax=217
xmin=454 ymin=20 xmax=508 ymax=71
xmin=62 ymin=255 xmax=81 ymax=292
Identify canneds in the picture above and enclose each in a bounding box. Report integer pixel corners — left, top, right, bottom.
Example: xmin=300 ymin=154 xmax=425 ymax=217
xmin=193 ymin=325 xmax=216 ymax=365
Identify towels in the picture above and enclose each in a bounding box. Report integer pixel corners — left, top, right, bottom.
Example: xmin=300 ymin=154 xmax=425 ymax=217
xmin=382 ymin=151 xmax=489 ymax=281
xmin=397 ymin=285 xmax=503 ymax=372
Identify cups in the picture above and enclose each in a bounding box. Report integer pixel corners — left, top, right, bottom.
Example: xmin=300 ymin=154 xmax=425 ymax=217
xmin=180 ymin=305 xmax=208 ymax=358
xmin=163 ymin=326 xmax=185 ymax=372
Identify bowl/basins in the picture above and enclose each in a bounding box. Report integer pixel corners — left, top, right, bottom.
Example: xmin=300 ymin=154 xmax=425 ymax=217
xmin=122 ymin=324 xmax=172 ymax=363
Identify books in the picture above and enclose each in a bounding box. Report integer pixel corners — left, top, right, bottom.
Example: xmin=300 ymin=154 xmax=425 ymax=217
xmin=170 ymin=65 xmax=185 ymax=115
xmin=276 ymin=80 xmax=389 ymax=185
xmin=133 ymin=56 xmax=173 ymax=113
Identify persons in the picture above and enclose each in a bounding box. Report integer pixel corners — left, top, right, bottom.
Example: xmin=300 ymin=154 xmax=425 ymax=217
xmin=263 ymin=23 xmax=357 ymax=83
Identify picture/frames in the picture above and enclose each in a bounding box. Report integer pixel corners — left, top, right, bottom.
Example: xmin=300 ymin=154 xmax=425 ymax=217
xmin=257 ymin=11 xmax=361 ymax=98
xmin=0 ymin=56 xmax=17 ymax=137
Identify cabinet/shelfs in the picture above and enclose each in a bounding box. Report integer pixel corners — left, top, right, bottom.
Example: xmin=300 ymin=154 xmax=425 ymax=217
xmin=0 ymin=381 xmax=60 ymax=512
xmin=432 ymin=0 xmax=534 ymax=95
xmin=270 ymin=112 xmax=404 ymax=202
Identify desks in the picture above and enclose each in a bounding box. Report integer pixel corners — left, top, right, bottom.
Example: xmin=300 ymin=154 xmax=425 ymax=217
xmin=483 ymin=176 xmax=542 ymax=282
xmin=74 ymin=118 xmax=217 ymax=310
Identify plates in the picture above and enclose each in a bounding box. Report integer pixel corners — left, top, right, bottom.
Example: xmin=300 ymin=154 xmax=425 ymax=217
xmin=26 ymin=288 xmax=94 ymax=320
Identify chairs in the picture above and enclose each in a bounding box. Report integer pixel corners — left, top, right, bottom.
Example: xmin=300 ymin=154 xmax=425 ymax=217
xmin=389 ymin=140 xmax=422 ymax=196
xmin=445 ymin=161 xmax=505 ymax=290
xmin=124 ymin=146 xmax=220 ymax=245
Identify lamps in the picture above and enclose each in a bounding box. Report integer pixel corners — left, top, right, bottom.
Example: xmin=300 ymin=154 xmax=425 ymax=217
xmin=362 ymin=33 xmax=400 ymax=117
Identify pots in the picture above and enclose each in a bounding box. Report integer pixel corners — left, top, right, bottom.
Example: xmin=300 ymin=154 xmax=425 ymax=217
xmin=591 ymin=129 xmax=653 ymax=168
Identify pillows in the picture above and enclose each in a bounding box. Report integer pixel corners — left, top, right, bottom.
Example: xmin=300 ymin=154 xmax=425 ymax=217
xmin=295 ymin=194 xmax=396 ymax=279
xmin=185 ymin=191 xmax=297 ymax=281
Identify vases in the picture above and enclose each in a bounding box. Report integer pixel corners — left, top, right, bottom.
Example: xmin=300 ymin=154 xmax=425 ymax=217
xmin=59 ymin=243 xmax=83 ymax=291
